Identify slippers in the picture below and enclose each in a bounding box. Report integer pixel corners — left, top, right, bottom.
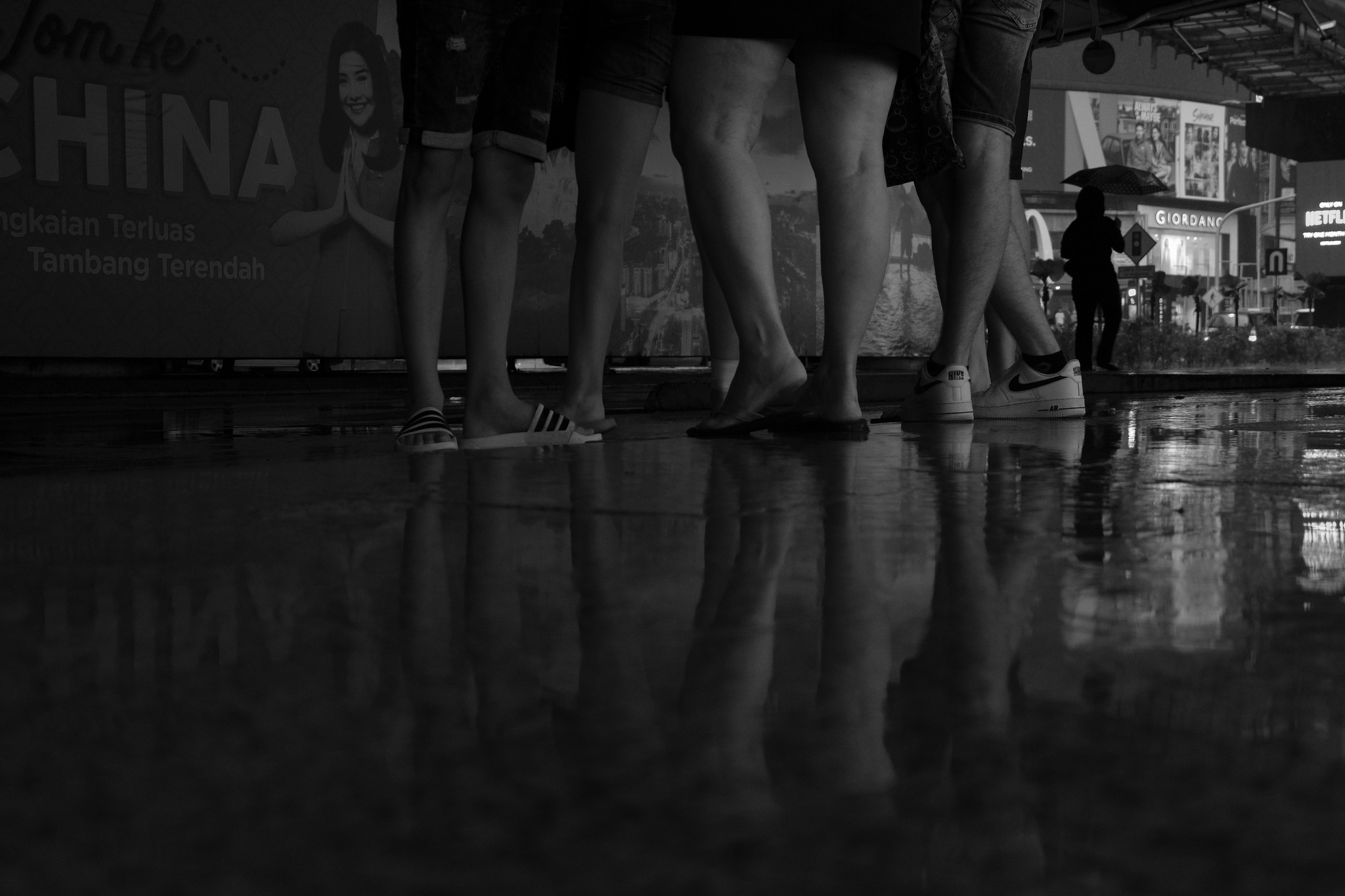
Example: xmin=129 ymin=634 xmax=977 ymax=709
xmin=394 ymin=407 xmax=460 ymax=455
xmin=686 ymin=407 xmax=821 ymax=440
xmin=458 ymin=402 xmax=604 ymax=450
xmin=768 ymin=420 xmax=871 ymax=442
xmin=574 ymin=418 xmax=618 ymax=435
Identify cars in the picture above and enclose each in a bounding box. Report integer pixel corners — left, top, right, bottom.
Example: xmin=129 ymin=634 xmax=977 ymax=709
xmin=184 ymin=356 xmax=409 ymax=380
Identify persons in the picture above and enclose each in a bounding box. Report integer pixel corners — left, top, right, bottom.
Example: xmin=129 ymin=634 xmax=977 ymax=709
xmin=30 ymin=402 xmax=1140 ymax=866
xmin=274 ymin=28 xmax=409 ymax=355
xmin=1124 ymin=122 xmax=1297 ymax=205
xmin=384 ymin=0 xmax=600 ymax=457
xmin=886 ymin=1 xmax=1088 ymax=420
xmin=882 ymin=0 xmax=1031 ymax=404
xmin=549 ymin=4 xmax=745 ymax=435
xmin=675 ymin=0 xmax=934 ymax=441
xmin=1059 ymin=186 xmax=1126 ymax=373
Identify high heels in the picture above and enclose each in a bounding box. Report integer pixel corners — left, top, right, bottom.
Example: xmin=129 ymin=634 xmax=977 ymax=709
xmin=1097 ymin=362 xmax=1121 ymax=371
xmin=1080 ymin=365 xmax=1093 ymax=370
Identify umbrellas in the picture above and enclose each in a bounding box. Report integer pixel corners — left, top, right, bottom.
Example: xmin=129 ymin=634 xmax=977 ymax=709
xmin=1057 ymin=163 xmax=1170 ymax=219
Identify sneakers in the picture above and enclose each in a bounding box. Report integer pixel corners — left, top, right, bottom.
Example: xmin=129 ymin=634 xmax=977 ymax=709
xmin=897 ymin=358 xmax=975 ymax=423
xmin=971 ymin=354 xmax=1086 ymax=420
